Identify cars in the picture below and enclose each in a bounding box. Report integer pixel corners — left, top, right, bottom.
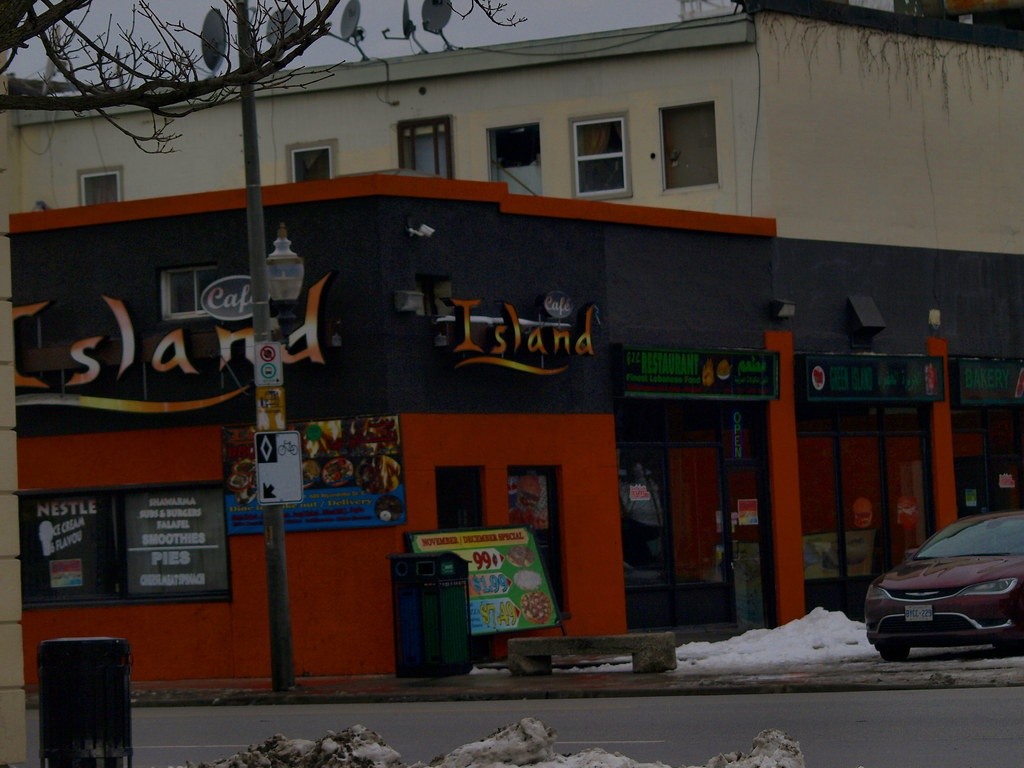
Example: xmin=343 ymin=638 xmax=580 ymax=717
xmin=863 ymin=508 xmax=1023 ymax=660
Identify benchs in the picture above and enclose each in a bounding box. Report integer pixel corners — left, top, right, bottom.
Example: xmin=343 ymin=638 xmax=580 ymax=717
xmin=507 ymin=632 xmax=677 ymax=675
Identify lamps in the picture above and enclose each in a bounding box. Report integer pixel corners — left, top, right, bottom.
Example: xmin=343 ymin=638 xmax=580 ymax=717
xmin=395 ymin=289 xmax=424 ymax=310
xmin=265 ymin=221 xmax=306 ymax=352
xmin=768 ymin=298 xmax=796 ymax=324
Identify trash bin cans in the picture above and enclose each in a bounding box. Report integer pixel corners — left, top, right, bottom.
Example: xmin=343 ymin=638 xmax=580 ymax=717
xmin=36 ymin=637 xmax=134 ymax=768
xmin=387 ymin=551 xmax=474 ymax=680
